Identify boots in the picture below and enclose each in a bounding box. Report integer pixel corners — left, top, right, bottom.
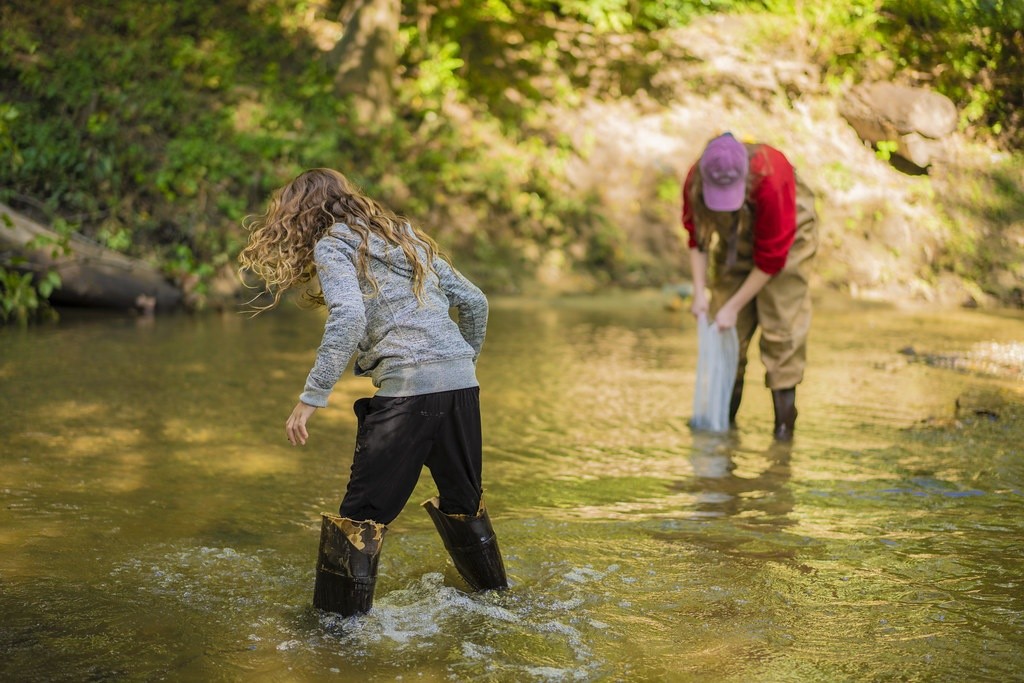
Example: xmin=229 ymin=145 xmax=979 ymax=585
xmin=422 ymin=489 xmax=508 ymax=591
xmin=314 ymin=510 xmax=387 ymax=618
xmin=773 ymin=386 xmax=796 ymax=439
xmin=730 ymin=380 xmax=743 ymax=419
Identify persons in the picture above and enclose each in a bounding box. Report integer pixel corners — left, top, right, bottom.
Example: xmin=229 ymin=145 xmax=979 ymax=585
xmin=236 ymin=168 xmax=509 ymax=614
xmin=682 ymin=133 xmax=820 ymax=431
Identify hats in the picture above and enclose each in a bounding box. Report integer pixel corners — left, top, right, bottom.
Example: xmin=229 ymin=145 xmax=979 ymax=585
xmin=699 ymin=136 xmax=747 ymax=211
